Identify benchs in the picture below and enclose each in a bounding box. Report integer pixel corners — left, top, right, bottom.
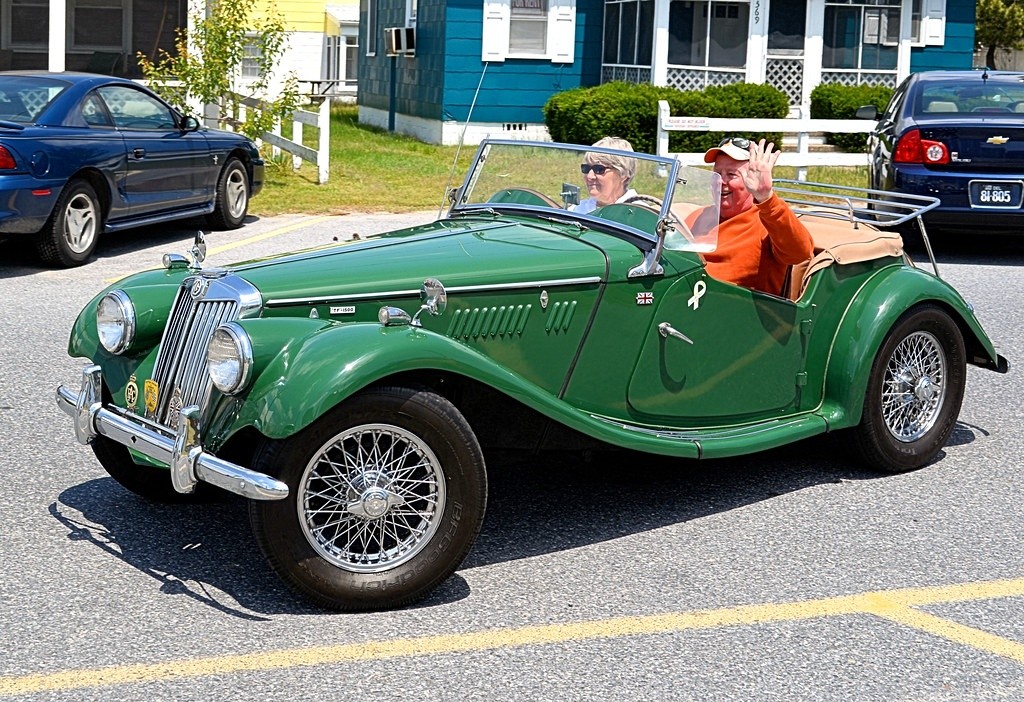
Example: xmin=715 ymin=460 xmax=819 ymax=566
xmin=655 ymin=202 xmax=875 ymax=302
xmin=294 ymin=93 xmax=358 ymax=98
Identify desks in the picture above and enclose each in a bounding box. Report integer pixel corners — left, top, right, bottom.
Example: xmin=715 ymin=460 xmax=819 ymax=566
xmin=296 ymin=78 xmax=358 ymax=106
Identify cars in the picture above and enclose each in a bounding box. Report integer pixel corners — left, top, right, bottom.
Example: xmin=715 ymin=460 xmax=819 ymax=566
xmin=0 ymin=72 xmax=266 ymax=271
xmin=56 ymin=137 xmax=1013 ymax=617
xmin=854 ymin=69 xmax=1024 ymax=251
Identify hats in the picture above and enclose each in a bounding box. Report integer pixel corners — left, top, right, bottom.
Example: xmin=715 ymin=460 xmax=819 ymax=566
xmin=704 ymin=138 xmax=758 ymax=163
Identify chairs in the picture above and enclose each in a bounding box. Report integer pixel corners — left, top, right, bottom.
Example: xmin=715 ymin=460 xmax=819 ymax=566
xmin=91 ymin=51 xmax=121 ymax=75
xmin=929 ymin=102 xmax=958 ymax=111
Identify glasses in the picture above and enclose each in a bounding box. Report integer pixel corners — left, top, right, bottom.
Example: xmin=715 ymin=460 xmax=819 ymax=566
xmin=580 ymin=164 xmax=614 ymax=175
xmin=718 ymin=137 xmax=750 ymax=152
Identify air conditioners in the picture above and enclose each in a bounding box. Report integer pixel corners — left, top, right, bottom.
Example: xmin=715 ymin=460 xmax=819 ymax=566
xmin=384 ymin=27 xmax=414 ymax=53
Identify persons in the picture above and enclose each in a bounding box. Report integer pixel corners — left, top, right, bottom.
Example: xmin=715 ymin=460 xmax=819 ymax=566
xmin=672 ymin=137 xmax=815 ymax=296
xmin=565 ymin=136 xmax=650 ymax=215
